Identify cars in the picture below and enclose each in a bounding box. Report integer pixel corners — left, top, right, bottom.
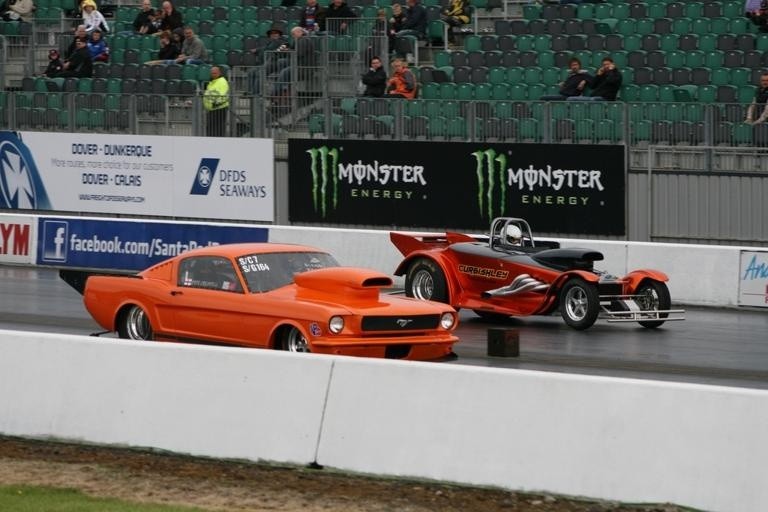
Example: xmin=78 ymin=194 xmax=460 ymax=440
xmin=59 ymin=241 xmax=461 ymax=362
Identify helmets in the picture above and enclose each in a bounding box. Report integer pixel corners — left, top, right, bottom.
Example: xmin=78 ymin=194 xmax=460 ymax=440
xmin=80 ymin=0 xmax=96 ymax=9
xmin=500 ymin=224 xmax=523 ymax=248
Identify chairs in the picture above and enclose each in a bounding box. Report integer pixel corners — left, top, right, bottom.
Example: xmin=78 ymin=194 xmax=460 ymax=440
xmin=0 ymin=0 xmax=768 ymax=149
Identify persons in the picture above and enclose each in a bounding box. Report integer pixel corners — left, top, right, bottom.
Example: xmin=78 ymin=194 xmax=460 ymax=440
xmin=265 ymin=26 xmax=317 ymax=104
xmin=384 ymin=56 xmax=418 ymax=99
xmin=1 ymin=0 xmax=207 ymax=80
xmin=352 ymin=55 xmax=386 ymax=116
xmin=744 ymin=0 xmax=767 ymax=34
xmin=742 ymin=72 xmax=767 ymax=146
xmin=297 ymin=0 xmax=327 ymax=37
xmin=588 ymin=57 xmax=623 ymax=101
xmin=388 ymin=0 xmax=409 ymax=49
xmin=424 ymin=0 xmax=473 ymax=48
xmin=358 ymin=7 xmax=396 ymax=66
xmin=318 ymin=0 xmax=359 ymax=37
xmin=539 ymin=57 xmax=596 ymax=99
xmin=242 ymin=20 xmax=289 ymax=96
xmin=200 ymin=65 xmax=232 ymax=137
xmin=498 ymin=223 xmax=523 ymax=249
xmin=394 ymin=0 xmax=424 ymax=51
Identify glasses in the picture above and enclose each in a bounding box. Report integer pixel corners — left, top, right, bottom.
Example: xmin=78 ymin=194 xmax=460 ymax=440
xmin=372 ymin=62 xmax=379 ymax=64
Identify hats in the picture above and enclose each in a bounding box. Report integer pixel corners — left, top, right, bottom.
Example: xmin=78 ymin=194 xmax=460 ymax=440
xmin=267 ymin=24 xmax=282 ymax=33
xmin=49 ymin=49 xmax=58 ymax=56
xmin=75 ymin=38 xmax=86 ymax=46
